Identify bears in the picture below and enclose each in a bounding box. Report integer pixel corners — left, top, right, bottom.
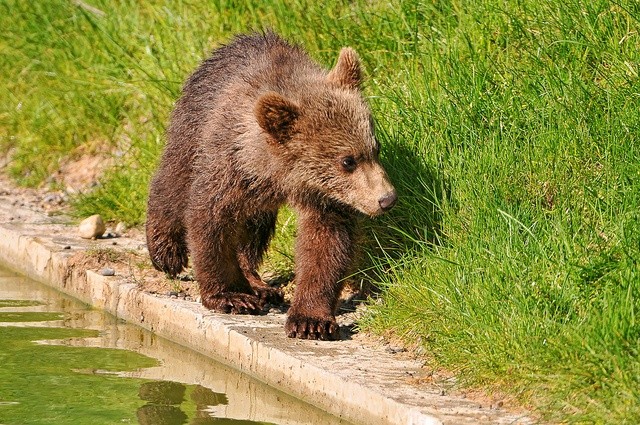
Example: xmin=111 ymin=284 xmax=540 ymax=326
xmin=145 ymin=26 xmax=399 ymax=341
